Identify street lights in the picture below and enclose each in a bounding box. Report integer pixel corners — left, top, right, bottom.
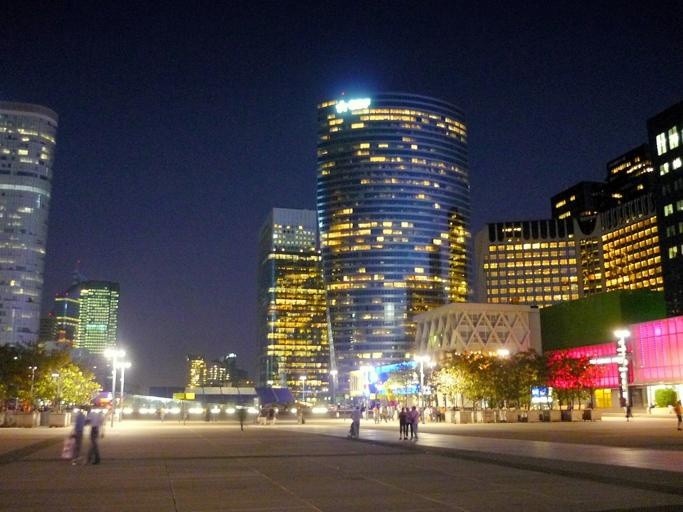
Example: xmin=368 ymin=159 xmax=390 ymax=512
xmin=613 ymin=327 xmax=631 ymax=417
xmin=99 ymin=346 xmax=132 ymax=428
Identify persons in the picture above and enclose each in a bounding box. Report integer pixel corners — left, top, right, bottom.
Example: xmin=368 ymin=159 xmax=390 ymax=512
xmin=236 ymin=406 xmax=247 ymax=431
xmin=296 ymin=405 xmax=303 ymax=425
xmin=256 ymin=406 xmax=279 ymax=425
xmin=371 ymin=404 xmax=394 ymax=424
xmin=397 ymin=407 xmax=407 ymax=441
xmin=349 ymin=406 xmax=362 ymax=440
xmin=404 ymin=407 xmax=414 ymax=439
xmin=66 ymin=406 xmax=86 ymax=467
xmin=415 ymin=405 xmax=443 ymax=425
xmin=83 ymin=404 xmax=104 ymax=466
xmin=410 ymin=406 xmax=420 ymax=440
xmin=674 ymin=400 xmax=683 ymax=431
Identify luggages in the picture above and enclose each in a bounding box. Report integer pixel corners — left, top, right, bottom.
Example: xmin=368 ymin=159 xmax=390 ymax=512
xmin=61 ymin=438 xmax=77 ymax=461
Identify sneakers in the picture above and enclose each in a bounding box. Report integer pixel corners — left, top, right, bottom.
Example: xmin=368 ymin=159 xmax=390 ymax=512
xmin=72 ymin=460 xmax=101 ymax=466
